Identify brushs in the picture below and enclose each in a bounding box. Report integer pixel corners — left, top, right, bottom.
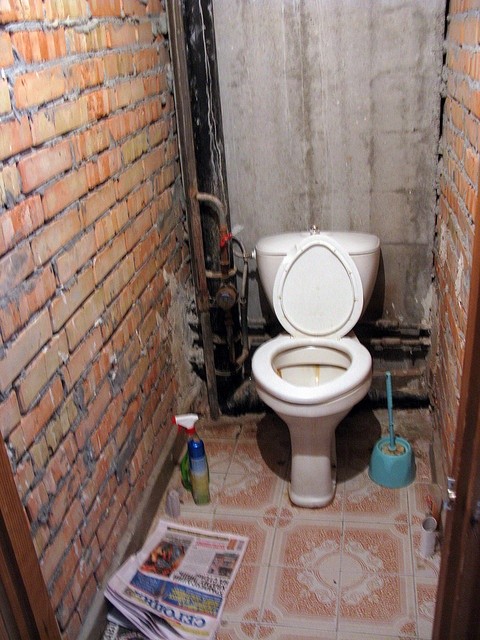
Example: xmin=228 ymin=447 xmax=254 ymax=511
xmin=378 ymin=372 xmax=405 ymax=454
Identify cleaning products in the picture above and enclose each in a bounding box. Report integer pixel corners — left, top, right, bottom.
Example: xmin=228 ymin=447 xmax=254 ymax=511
xmin=170 ymin=414 xmax=205 ymax=490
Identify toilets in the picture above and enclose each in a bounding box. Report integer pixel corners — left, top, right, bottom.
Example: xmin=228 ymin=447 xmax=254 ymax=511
xmin=251 ymin=231 xmax=381 ymax=507
